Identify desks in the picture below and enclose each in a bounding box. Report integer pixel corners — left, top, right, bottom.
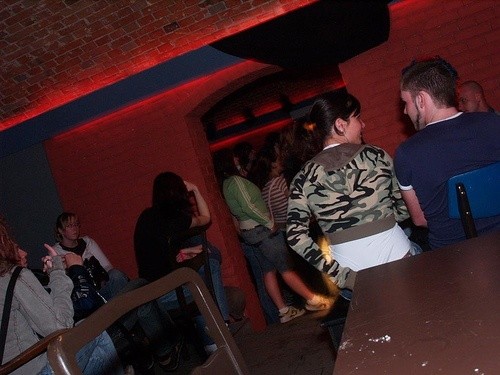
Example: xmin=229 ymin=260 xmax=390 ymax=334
xmin=332 ymin=232 xmax=500 ymax=375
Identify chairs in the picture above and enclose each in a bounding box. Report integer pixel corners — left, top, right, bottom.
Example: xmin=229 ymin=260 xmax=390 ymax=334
xmin=47 ymin=268 xmax=251 ymax=375
xmin=167 ymin=226 xmax=224 ymax=362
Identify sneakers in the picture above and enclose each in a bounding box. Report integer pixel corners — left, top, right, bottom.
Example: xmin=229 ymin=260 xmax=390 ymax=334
xmin=305 ymin=294 xmax=337 ymax=311
xmin=278 ymin=305 xmax=306 ymax=324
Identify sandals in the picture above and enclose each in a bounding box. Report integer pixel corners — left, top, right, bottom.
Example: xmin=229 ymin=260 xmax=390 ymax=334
xmin=156 ymin=335 xmax=184 ymax=371
xmin=143 ymin=351 xmax=153 ymax=371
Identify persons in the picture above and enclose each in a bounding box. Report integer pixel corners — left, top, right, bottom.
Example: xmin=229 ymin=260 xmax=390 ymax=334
xmin=176 ymin=190 xmax=222 ymax=265
xmin=279 ymin=89 xmax=424 ymax=301
xmin=26 ymin=245 xmax=185 ymax=371
xmin=0 ymin=215 xmax=134 ymax=375
xmin=251 ymin=148 xmax=330 ymax=295
xmin=133 ymin=172 xmax=239 ymax=336
xmin=219 ymin=151 xmax=337 ymax=323
xmin=48 ymin=212 xmax=130 ymax=301
xmin=459 ymin=80 xmax=500 ymax=115
xmin=393 ymin=55 xmax=500 ymax=250
